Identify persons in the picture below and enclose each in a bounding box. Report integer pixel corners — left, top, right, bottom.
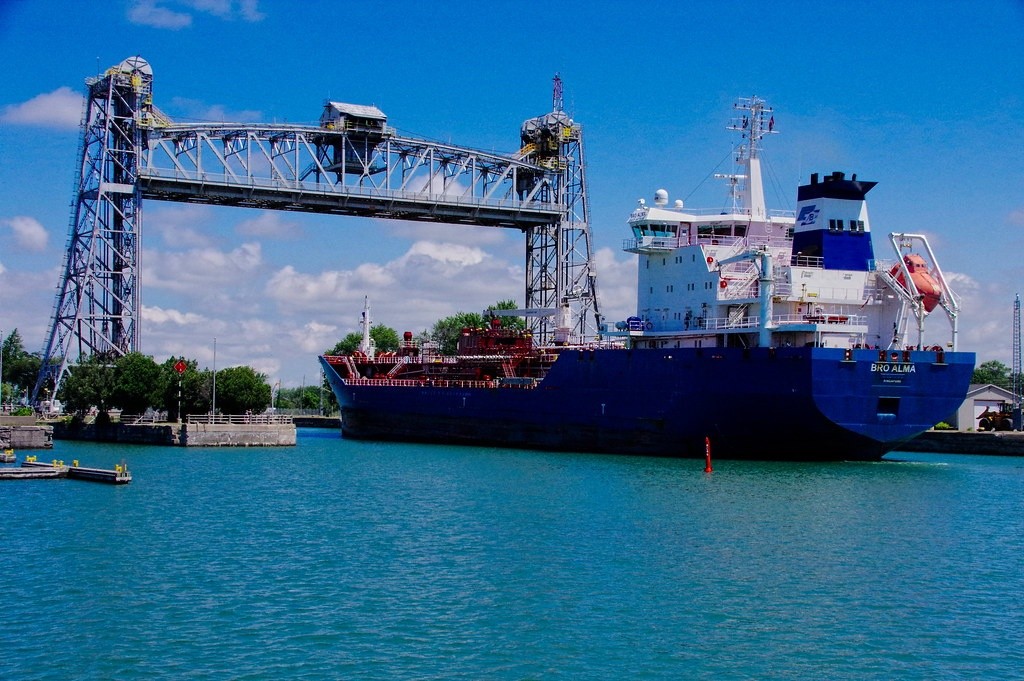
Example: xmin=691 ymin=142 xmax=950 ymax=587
xmin=491 ymin=315 xmax=501 ymax=328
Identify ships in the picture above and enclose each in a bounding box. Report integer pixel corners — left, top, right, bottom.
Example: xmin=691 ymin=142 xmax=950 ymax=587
xmin=315 ymin=91 xmax=977 ymax=460
